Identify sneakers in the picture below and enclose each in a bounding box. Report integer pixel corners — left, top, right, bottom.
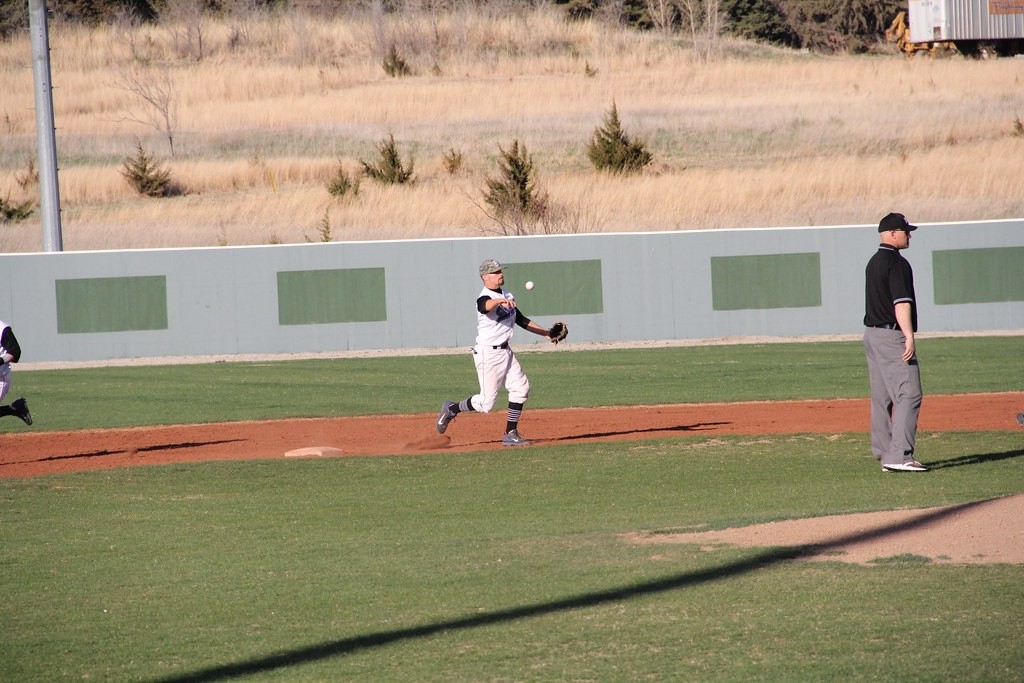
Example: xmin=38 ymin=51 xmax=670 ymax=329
xmin=437 ymin=400 xmax=457 ymax=434
xmin=13 ymin=397 xmax=32 ymax=426
xmin=502 ymin=428 xmax=535 ymax=446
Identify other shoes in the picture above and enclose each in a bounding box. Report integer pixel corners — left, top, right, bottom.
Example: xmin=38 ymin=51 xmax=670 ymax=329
xmin=882 ymin=460 xmax=932 ymax=472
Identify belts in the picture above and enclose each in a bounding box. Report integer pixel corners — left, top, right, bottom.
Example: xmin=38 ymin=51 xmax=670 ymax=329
xmin=868 ymin=323 xmax=903 ymax=331
xmin=476 ymin=342 xmax=509 ymax=350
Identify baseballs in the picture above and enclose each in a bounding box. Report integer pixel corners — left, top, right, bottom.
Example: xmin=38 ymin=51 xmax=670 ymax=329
xmin=525 ymin=281 xmax=534 ymax=290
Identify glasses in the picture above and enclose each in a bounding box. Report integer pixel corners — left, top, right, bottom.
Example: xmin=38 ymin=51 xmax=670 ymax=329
xmin=890 ymin=229 xmax=910 ymax=237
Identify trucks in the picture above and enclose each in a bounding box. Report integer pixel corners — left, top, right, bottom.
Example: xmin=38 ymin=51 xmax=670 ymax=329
xmin=909 ymin=1 xmax=1024 ymax=58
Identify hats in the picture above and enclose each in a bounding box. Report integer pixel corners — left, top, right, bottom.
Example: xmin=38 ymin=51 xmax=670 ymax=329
xmin=479 ymin=259 xmax=508 ymax=277
xmin=878 ymin=213 xmax=918 ymax=233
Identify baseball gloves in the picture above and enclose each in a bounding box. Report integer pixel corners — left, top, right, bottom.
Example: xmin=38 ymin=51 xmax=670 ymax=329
xmin=549 ymin=322 xmax=568 ymax=343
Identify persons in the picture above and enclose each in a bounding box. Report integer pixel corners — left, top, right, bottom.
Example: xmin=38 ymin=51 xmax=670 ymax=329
xmin=863 ymin=212 xmax=928 ymax=473
xmin=437 ymin=260 xmax=569 ymax=446
xmin=0 ymin=320 xmax=33 ymax=426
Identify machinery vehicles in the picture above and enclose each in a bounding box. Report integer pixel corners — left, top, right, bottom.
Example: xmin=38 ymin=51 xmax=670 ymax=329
xmin=886 ymin=12 xmax=996 ymax=60
